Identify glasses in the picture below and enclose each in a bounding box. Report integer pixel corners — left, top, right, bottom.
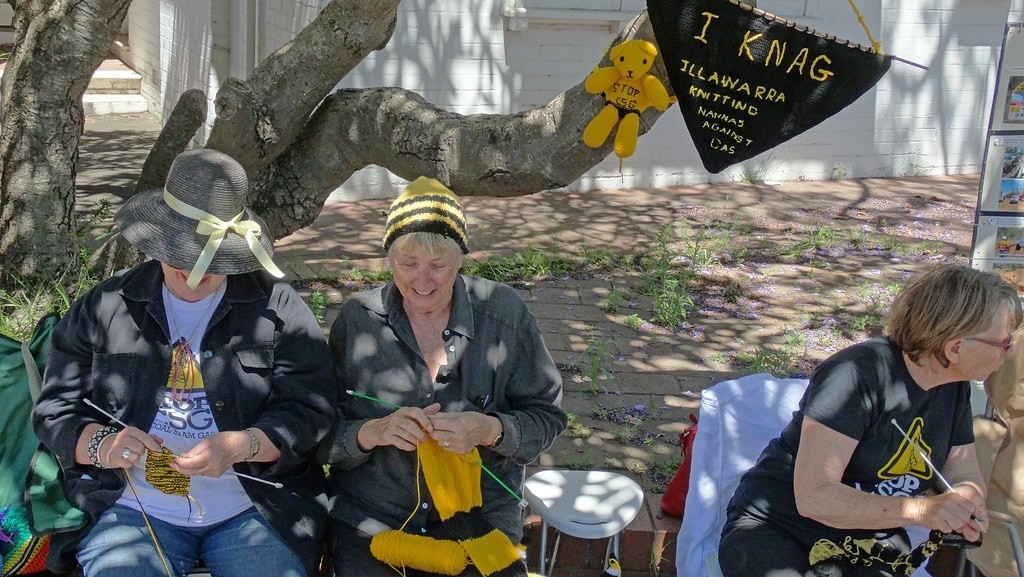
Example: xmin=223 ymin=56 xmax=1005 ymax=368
xmin=961 ymin=333 xmax=1014 ymax=353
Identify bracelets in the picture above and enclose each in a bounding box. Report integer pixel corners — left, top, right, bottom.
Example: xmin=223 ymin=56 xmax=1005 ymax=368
xmin=88 ymin=426 xmax=119 ymax=469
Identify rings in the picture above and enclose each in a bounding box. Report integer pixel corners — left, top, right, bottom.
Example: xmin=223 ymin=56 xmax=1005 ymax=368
xmin=123 ymin=449 xmax=132 ymax=459
xmin=444 ymin=441 xmax=449 ymax=447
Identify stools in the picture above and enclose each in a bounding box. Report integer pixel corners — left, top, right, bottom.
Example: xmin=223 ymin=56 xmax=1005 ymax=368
xmin=522 ymin=471 xmax=657 ymax=577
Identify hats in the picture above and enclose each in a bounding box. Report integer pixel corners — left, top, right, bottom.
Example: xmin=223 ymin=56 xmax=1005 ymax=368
xmin=113 ymin=148 xmax=286 ymax=291
xmin=383 ymin=176 xmax=471 ymax=254
xmin=0 ymin=505 xmax=51 ymax=577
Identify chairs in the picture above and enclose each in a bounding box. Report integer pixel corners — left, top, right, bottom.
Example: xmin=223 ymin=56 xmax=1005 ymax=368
xmin=674 ymin=373 xmax=934 ymax=576
xmin=0 ymin=311 xmax=87 ymax=577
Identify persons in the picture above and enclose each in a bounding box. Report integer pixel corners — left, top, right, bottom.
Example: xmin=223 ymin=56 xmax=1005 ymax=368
xmin=308 ymin=176 xmax=567 ymax=577
xmin=31 ymin=150 xmax=338 ymax=577
xmin=998 ymin=236 xmax=1022 ymax=258
xmin=718 ymin=266 xmax=1024 ymax=577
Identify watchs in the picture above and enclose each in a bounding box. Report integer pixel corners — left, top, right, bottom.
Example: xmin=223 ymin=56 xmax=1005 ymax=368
xmin=243 ymin=430 xmax=260 ymax=461
xmin=489 ymin=418 xmax=504 ymax=448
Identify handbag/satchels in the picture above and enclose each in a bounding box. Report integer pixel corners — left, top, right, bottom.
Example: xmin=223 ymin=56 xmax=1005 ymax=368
xmin=0 ymin=313 xmax=89 ymax=537
xmin=661 ymin=412 xmax=697 ymax=518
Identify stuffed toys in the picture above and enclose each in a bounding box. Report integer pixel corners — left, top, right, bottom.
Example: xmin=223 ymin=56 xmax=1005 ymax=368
xmin=583 ymin=40 xmax=669 ymax=172
